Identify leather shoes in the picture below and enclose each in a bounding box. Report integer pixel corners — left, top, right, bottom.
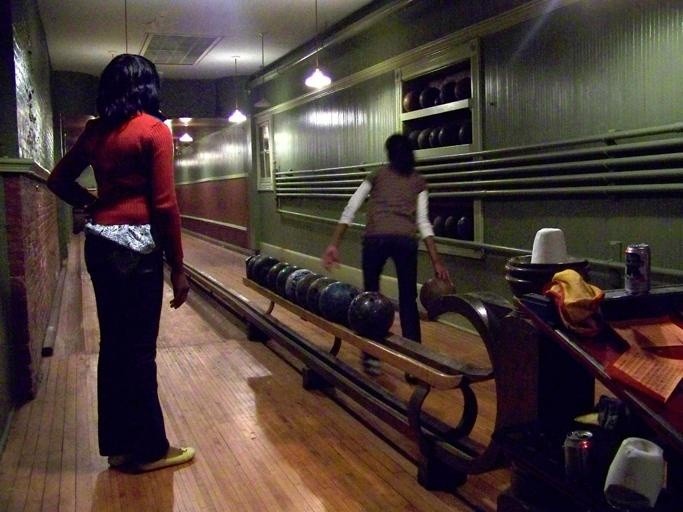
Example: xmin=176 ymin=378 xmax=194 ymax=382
xmin=105 ymin=444 xmax=196 ymax=472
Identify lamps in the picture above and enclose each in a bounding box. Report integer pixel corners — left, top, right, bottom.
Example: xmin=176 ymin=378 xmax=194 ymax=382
xmin=304 ymin=2 xmax=333 ymax=89
xmin=228 ymin=29 xmax=271 ymax=125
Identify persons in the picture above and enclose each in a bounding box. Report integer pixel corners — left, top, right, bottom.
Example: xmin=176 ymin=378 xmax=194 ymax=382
xmin=48 ymin=54 xmax=195 ymax=472
xmin=322 ymin=135 xmax=454 ymax=384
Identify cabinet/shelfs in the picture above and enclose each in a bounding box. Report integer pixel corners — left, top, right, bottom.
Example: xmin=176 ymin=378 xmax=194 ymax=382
xmin=394 ymin=39 xmax=484 ymax=244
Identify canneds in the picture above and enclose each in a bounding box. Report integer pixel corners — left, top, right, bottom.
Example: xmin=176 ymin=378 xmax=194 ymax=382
xmin=624 ymin=242 xmax=652 ymax=295
xmin=564 ymin=430 xmax=598 ymax=484
